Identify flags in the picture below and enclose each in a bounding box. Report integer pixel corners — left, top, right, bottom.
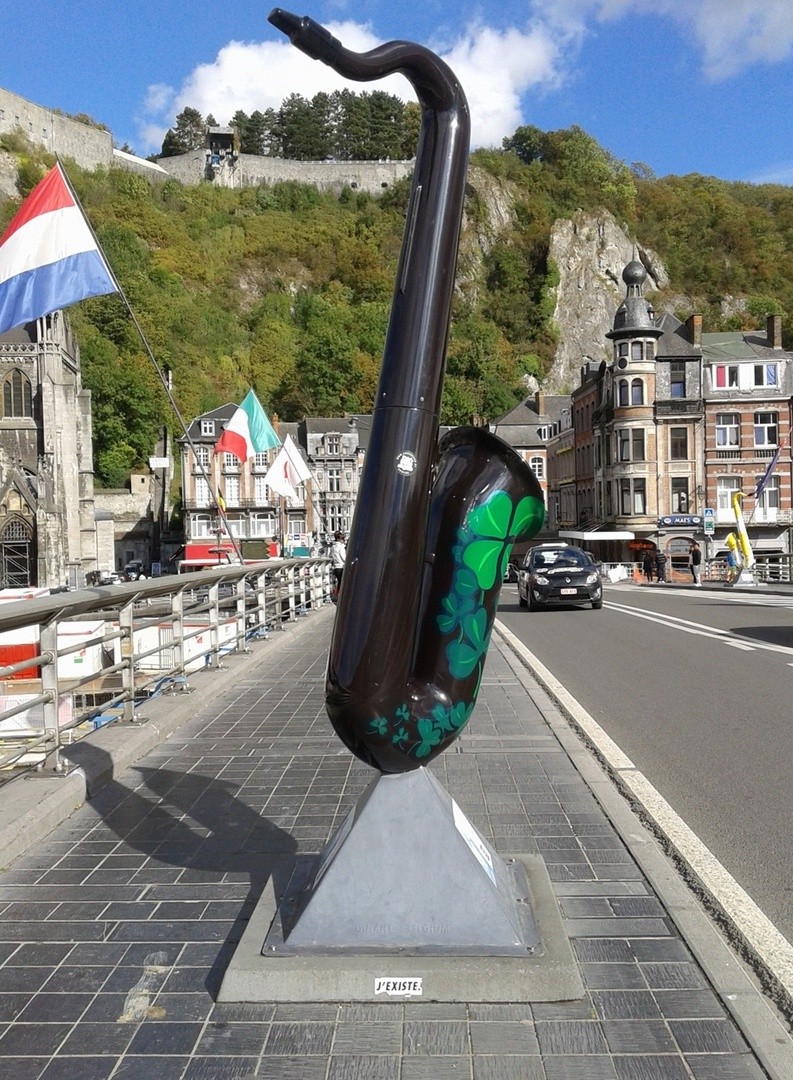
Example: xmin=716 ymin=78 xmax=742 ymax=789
xmin=213 ymin=386 xmax=282 ymax=464
xmin=263 ymin=431 xmax=312 ymax=502
xmin=0 ymin=162 xmax=119 ymax=335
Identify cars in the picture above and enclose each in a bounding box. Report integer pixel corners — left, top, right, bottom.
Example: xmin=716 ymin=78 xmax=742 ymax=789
xmin=288 ymin=530 xmax=350 ymax=549
xmin=584 ymin=551 xmax=603 ymax=576
xmin=49 ymin=558 xmax=144 ymax=595
xmin=502 ymin=563 xmax=520 ymax=583
xmin=510 ymin=544 xmax=603 ymax=612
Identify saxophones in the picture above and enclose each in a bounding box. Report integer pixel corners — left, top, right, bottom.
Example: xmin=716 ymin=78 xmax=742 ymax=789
xmin=264 ymin=6 xmax=548 ymax=775
xmin=724 ymin=492 xmax=754 ymax=569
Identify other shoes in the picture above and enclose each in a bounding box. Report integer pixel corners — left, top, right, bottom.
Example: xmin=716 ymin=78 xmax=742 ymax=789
xmin=659 ymin=581 xmax=662 ymax=583
xmin=695 ymin=583 xmax=702 ymax=587
xmin=662 ymin=581 xmax=665 ymax=583
xmin=648 ymin=582 xmax=651 ymax=584
xmin=651 ymin=582 xmax=654 ymax=584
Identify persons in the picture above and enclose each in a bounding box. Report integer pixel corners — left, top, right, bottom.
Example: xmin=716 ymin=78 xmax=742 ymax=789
xmin=329 ymin=533 xmax=346 ymax=605
xmin=655 ymin=550 xmax=667 ymax=583
xmin=688 ymin=543 xmax=702 ymax=586
xmin=643 ymin=550 xmax=655 ymax=584
